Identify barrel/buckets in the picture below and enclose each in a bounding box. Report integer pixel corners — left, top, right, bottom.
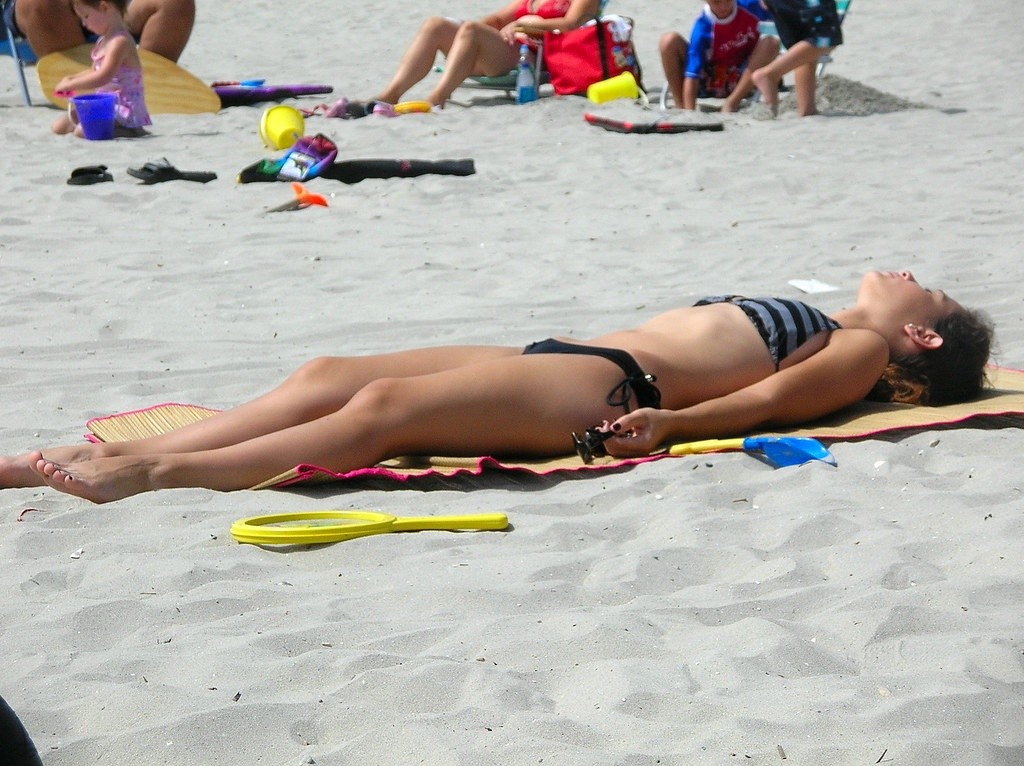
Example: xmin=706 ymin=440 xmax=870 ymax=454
xmin=68 ymin=94 xmax=116 ymax=140
xmin=587 ymin=71 xmax=649 ymax=107
xmin=259 ymin=105 xmax=305 ymax=151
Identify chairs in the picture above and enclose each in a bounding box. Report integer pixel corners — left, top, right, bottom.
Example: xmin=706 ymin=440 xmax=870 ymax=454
xmin=433 ymin=0 xmax=608 ymax=110
xmin=660 ymin=0 xmax=852 ymax=110
xmin=0 ymin=26 xmax=140 ymax=108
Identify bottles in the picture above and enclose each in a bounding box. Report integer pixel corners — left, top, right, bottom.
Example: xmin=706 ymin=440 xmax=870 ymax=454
xmin=519 ymin=63 xmax=534 ymax=104
xmin=516 ymin=44 xmax=534 ymax=105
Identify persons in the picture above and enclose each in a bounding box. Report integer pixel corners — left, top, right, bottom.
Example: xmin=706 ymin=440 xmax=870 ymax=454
xmin=0 ymin=0 xmax=195 ymax=63
xmin=751 ymin=0 xmax=843 ymax=116
xmin=52 ymin=0 xmax=153 ymax=138
xmin=356 ymin=0 xmax=601 ymax=108
xmin=0 ymin=273 xmax=992 ymax=505
xmin=659 ymin=0 xmax=780 ymax=111
